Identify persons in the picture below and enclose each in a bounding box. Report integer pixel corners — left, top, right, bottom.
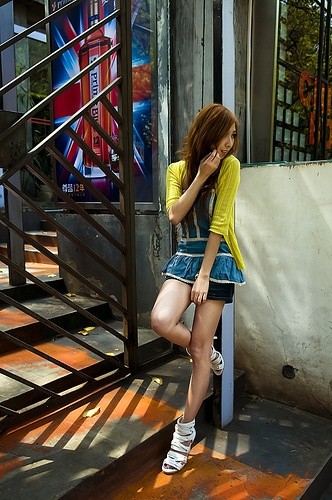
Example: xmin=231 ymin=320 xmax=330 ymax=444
xmin=151 ymin=105 xmax=246 ymax=475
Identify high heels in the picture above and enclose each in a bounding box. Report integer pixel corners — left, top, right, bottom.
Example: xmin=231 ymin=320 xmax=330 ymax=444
xmin=184 ymin=335 xmax=225 ymax=376
xmin=161 ymin=416 xmax=197 ymax=474
